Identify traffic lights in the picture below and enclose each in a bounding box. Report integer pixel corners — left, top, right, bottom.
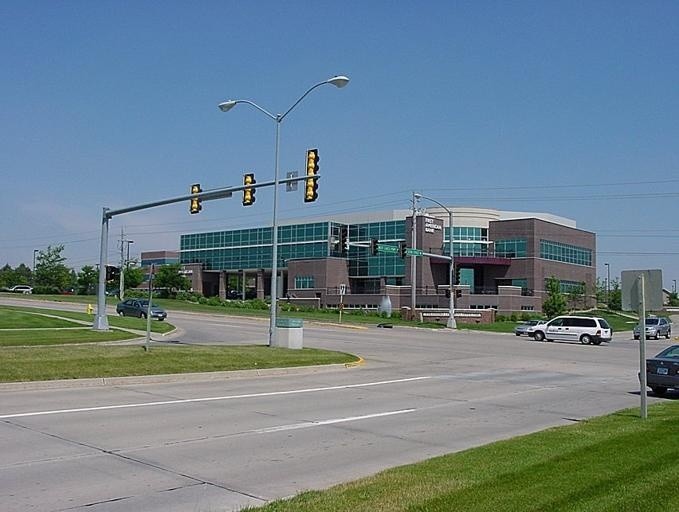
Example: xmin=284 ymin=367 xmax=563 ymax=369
xmin=338 ymin=222 xmax=408 ymax=260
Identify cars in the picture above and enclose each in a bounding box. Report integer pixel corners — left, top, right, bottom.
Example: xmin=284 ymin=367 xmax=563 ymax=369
xmin=525 ymin=315 xmax=613 ymax=345
xmin=637 ymin=343 xmax=678 ymax=395
xmin=116 ymin=297 xmax=168 ymax=322
xmin=633 ymin=316 xmax=672 ymax=340
xmin=515 ymin=318 xmax=548 ymax=336
xmin=9 ymin=284 xmax=34 ymax=296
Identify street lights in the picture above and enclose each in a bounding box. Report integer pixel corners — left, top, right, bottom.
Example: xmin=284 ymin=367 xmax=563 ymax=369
xmin=216 ymin=76 xmax=350 ymax=347
xmin=413 ymin=193 xmax=458 ymax=331
